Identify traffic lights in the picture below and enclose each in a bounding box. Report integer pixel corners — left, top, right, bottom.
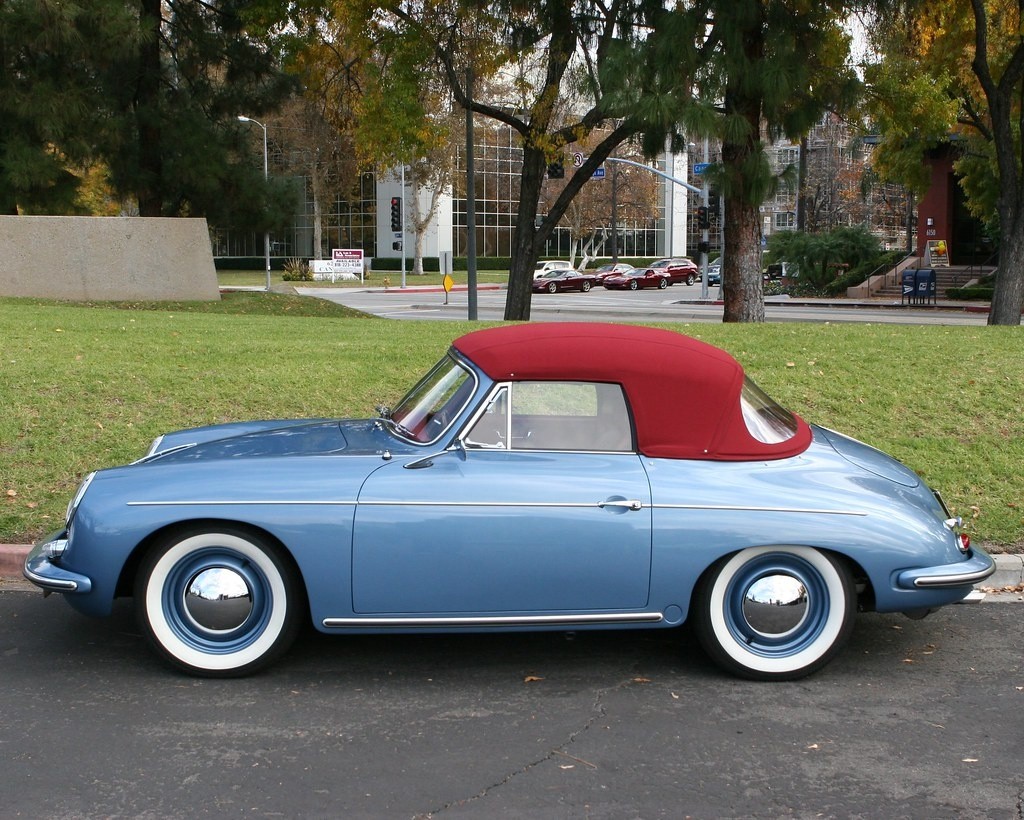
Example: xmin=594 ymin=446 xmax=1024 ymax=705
xmin=697 ymin=207 xmax=710 ymax=229
xmin=548 ymin=152 xmax=563 ymax=178
xmin=391 ymin=197 xmax=403 ymax=232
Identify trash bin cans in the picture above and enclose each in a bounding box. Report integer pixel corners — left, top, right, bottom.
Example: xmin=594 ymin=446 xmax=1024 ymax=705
xmin=901 ymin=269 xmax=937 ymax=305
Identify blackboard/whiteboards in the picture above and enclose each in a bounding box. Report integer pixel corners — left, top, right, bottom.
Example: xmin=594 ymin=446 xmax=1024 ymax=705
xmin=927 ymin=240 xmax=949 ymax=266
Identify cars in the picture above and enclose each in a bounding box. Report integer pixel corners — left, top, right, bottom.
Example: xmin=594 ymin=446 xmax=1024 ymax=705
xmin=588 ymin=263 xmax=634 ymax=286
xmin=604 ymin=267 xmax=671 ymax=291
xmin=21 ymin=322 xmax=996 ymax=679
xmin=532 ymin=269 xmax=598 ymax=293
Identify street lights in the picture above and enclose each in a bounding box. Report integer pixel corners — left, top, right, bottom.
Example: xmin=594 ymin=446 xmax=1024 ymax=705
xmin=238 ymin=116 xmax=271 ymax=290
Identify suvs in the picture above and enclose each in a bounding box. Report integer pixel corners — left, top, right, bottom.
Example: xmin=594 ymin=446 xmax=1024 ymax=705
xmin=700 ymin=257 xmax=722 ymax=286
xmin=646 ymin=258 xmax=698 ymax=286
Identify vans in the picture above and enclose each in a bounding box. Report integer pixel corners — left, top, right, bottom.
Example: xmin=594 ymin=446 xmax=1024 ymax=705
xmin=533 ymin=261 xmax=572 ymax=282
